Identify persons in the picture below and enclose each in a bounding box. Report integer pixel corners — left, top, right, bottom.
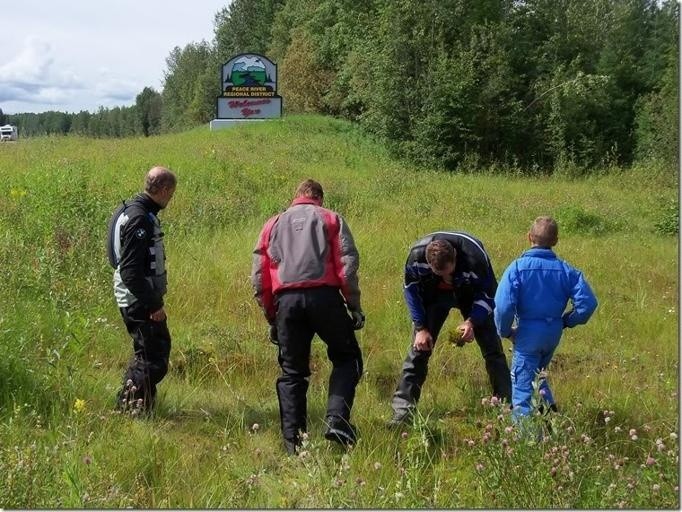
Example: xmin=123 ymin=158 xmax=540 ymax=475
xmin=495 ymin=216 xmax=598 ymax=427
xmin=388 ymin=231 xmax=511 ymax=429
xmin=253 ymin=177 xmax=365 ymax=460
xmin=107 ymin=167 xmax=177 ymax=418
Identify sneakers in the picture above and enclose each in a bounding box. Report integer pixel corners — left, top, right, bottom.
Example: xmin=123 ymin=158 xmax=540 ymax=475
xmin=388 ymin=412 xmax=418 ymax=433
xmin=324 ymin=416 xmax=359 ymax=446
